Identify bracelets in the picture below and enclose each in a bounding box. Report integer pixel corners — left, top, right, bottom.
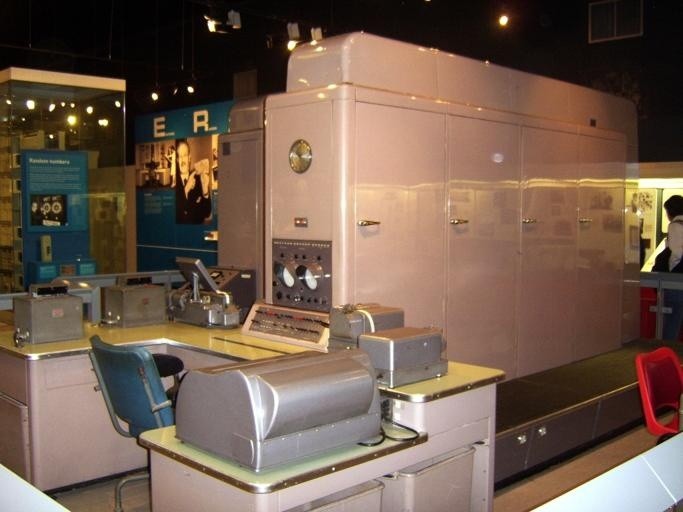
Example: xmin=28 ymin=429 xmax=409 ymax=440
xmin=203 ymin=191 xmax=209 ymax=196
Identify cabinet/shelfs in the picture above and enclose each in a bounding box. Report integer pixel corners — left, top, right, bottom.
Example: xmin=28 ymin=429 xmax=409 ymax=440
xmin=264 ymin=83 xmax=628 ymax=382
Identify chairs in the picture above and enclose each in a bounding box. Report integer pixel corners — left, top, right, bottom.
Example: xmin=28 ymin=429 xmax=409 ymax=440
xmin=633 ymin=345 xmax=683 ymax=443
xmin=86 ymin=332 xmax=175 ymax=511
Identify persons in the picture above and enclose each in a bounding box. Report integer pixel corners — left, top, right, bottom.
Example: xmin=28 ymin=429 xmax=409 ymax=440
xmin=167 ymin=145 xmax=176 ymax=188
xmin=664 ymin=195 xmax=683 ymax=273
xmin=176 ymin=140 xmax=211 ymax=224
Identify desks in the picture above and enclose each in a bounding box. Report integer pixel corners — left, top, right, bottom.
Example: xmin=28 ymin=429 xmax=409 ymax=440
xmin=0 ymin=317 xmax=506 ymax=511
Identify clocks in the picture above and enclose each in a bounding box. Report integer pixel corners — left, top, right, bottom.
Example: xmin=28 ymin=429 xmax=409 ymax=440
xmin=288 ymin=140 xmax=312 ymax=174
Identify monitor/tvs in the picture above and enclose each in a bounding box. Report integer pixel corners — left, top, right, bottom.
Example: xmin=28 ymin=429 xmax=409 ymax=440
xmin=175 ymin=257 xmax=218 ymax=293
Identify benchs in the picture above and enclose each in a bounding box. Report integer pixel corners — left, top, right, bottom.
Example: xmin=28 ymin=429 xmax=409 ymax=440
xmin=492 ymin=337 xmax=683 ymax=488
